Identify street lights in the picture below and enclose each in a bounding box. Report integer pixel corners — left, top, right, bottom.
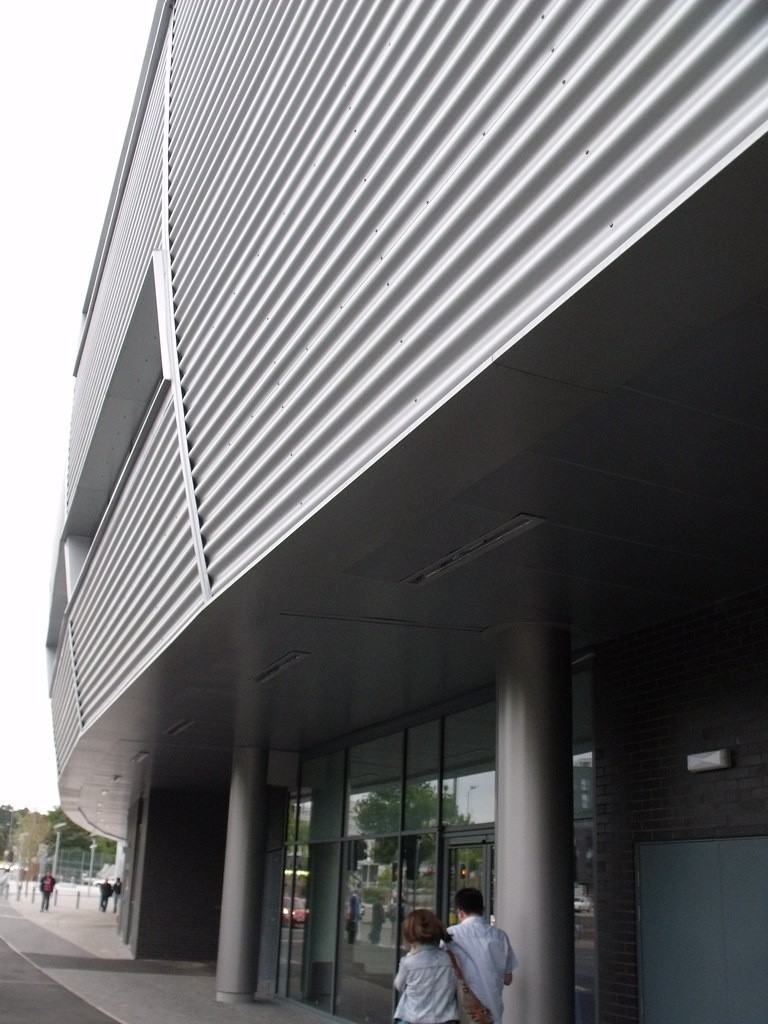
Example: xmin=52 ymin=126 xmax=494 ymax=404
xmin=87 ymin=833 xmax=96 ymax=898
xmin=90 ymin=844 xmax=96 ymax=886
xmin=52 ymin=823 xmax=66 ymax=877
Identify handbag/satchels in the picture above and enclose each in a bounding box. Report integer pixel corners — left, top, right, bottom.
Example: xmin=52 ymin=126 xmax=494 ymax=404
xmin=461 ymin=986 xmax=493 ymax=1022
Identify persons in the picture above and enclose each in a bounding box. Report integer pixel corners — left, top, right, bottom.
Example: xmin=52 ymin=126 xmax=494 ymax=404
xmin=40 ymin=870 xmax=58 ymax=912
xmin=99 ymin=878 xmax=114 ymax=912
xmin=392 ymin=909 xmax=462 ymax=1024
xmin=437 ymin=886 xmax=520 ymax=1024
xmin=389 ymin=897 xmax=408 ymax=946
xmin=113 ymin=877 xmax=122 ymax=913
xmin=368 ymin=896 xmax=386 ymax=944
xmin=346 ymin=888 xmax=362 ymax=944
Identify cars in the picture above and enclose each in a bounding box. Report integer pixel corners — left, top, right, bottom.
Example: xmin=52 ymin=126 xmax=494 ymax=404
xmin=280 ymin=897 xmax=306 ymax=929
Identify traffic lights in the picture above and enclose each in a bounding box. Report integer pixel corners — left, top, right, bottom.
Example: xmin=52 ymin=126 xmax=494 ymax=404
xmin=392 ymin=861 xmax=399 ymax=881
xmin=460 ymin=864 xmax=467 ymax=880
